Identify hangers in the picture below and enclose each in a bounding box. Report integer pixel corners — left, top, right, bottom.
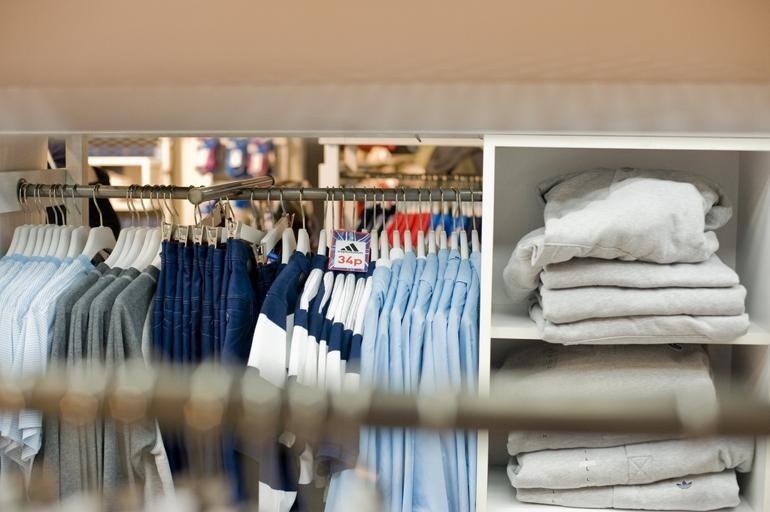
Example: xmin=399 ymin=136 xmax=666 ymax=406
xmin=5 ymin=180 xmax=482 ymax=265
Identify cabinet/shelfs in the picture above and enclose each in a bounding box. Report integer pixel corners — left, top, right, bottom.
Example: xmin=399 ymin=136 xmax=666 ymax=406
xmin=318 ymin=137 xmax=483 ymax=249
xmin=476 ymin=133 xmax=770 ymax=512
